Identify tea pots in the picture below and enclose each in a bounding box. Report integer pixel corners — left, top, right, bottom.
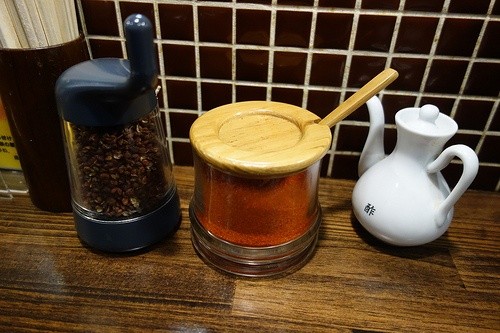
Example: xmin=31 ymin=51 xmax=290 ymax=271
xmin=351 ymin=95 xmax=479 ymax=247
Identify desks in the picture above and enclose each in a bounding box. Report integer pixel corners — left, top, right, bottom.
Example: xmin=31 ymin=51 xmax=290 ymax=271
xmin=0 ymin=164 xmax=499 ymax=333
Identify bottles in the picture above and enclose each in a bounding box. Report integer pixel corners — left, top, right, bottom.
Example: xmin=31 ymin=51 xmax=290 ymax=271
xmin=188 ymin=100 xmax=333 ymax=277
xmin=53 ymin=14 xmax=182 ymax=255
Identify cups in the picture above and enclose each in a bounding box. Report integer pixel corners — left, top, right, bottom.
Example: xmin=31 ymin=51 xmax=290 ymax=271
xmin=0 ymin=31 xmax=92 ymax=212
